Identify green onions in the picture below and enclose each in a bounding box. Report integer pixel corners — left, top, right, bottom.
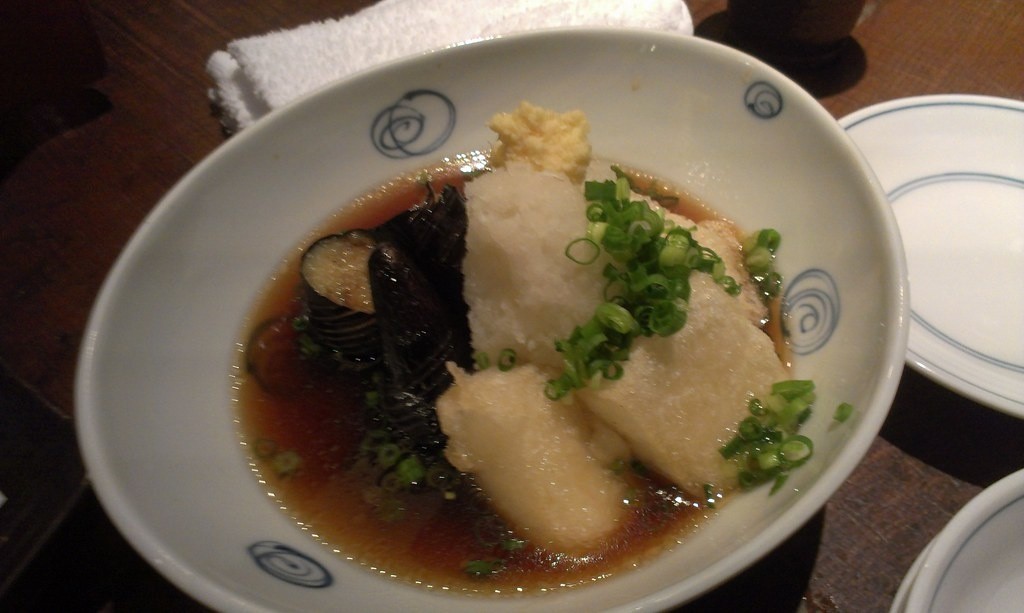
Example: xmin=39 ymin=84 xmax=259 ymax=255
xmin=245 ymin=161 xmax=853 ymax=584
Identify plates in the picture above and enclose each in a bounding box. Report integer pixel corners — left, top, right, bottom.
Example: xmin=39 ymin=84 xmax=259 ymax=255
xmin=835 ymin=93 xmax=1024 ymax=419
xmin=886 ymin=529 xmax=940 ymax=613
xmin=75 ymin=25 xmax=909 ymax=613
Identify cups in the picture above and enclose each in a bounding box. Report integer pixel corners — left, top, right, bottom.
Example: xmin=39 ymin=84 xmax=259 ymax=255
xmin=726 ymin=0 xmax=865 ymax=52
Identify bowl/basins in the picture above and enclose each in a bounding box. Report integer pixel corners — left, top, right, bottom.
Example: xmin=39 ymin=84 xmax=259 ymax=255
xmin=905 ymin=467 xmax=1024 ymax=613
